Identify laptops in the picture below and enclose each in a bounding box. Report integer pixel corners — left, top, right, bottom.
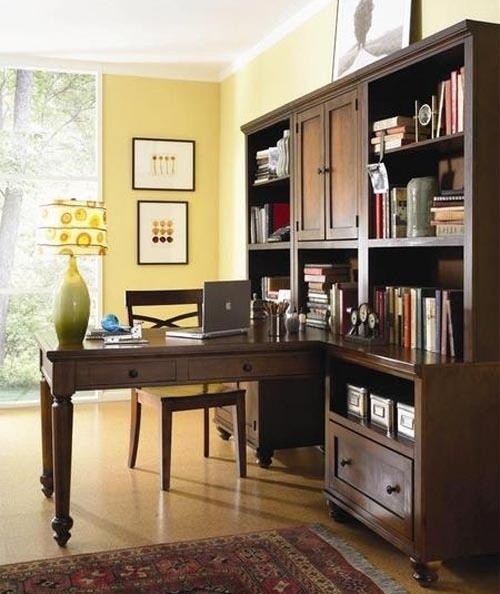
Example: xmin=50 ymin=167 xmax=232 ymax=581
xmin=165 ymin=280 xmax=251 ymax=339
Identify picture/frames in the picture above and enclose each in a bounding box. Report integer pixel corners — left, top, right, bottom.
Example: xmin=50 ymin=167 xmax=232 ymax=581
xmin=136 ymin=199 xmax=189 ymax=265
xmin=131 ymin=137 xmax=197 ymax=193
xmin=330 ymin=1 xmax=415 ymax=82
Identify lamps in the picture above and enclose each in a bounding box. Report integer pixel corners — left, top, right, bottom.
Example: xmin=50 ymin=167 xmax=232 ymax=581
xmin=37 ymin=197 xmax=109 ymax=346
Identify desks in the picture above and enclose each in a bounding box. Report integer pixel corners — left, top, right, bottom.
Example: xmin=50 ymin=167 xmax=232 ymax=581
xmin=37 ymin=327 xmax=331 ymax=547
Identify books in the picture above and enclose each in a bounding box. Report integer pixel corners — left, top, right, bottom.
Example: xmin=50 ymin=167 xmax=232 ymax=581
xmin=249 ymin=126 xmax=291 ymax=243
xmin=370 ymin=67 xmax=464 ymax=239
xmin=261 ymin=261 xmax=463 ymax=358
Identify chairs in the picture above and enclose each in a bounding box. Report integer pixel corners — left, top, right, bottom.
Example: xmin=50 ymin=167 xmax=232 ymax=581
xmin=123 ymin=288 xmax=248 ymax=490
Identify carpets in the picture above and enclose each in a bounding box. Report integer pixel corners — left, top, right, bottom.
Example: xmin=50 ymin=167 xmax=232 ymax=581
xmin=2 ymin=523 xmax=407 ymax=593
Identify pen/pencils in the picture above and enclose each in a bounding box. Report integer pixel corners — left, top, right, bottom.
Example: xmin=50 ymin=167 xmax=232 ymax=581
xmin=119 ymin=338 xmax=145 ymax=341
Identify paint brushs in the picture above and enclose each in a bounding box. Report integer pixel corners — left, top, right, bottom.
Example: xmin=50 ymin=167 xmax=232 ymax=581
xmin=262 ymin=299 xmax=289 ymax=315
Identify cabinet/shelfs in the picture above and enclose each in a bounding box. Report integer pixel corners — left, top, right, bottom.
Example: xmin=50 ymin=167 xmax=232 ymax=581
xmin=215 ymin=17 xmax=498 ymax=589
